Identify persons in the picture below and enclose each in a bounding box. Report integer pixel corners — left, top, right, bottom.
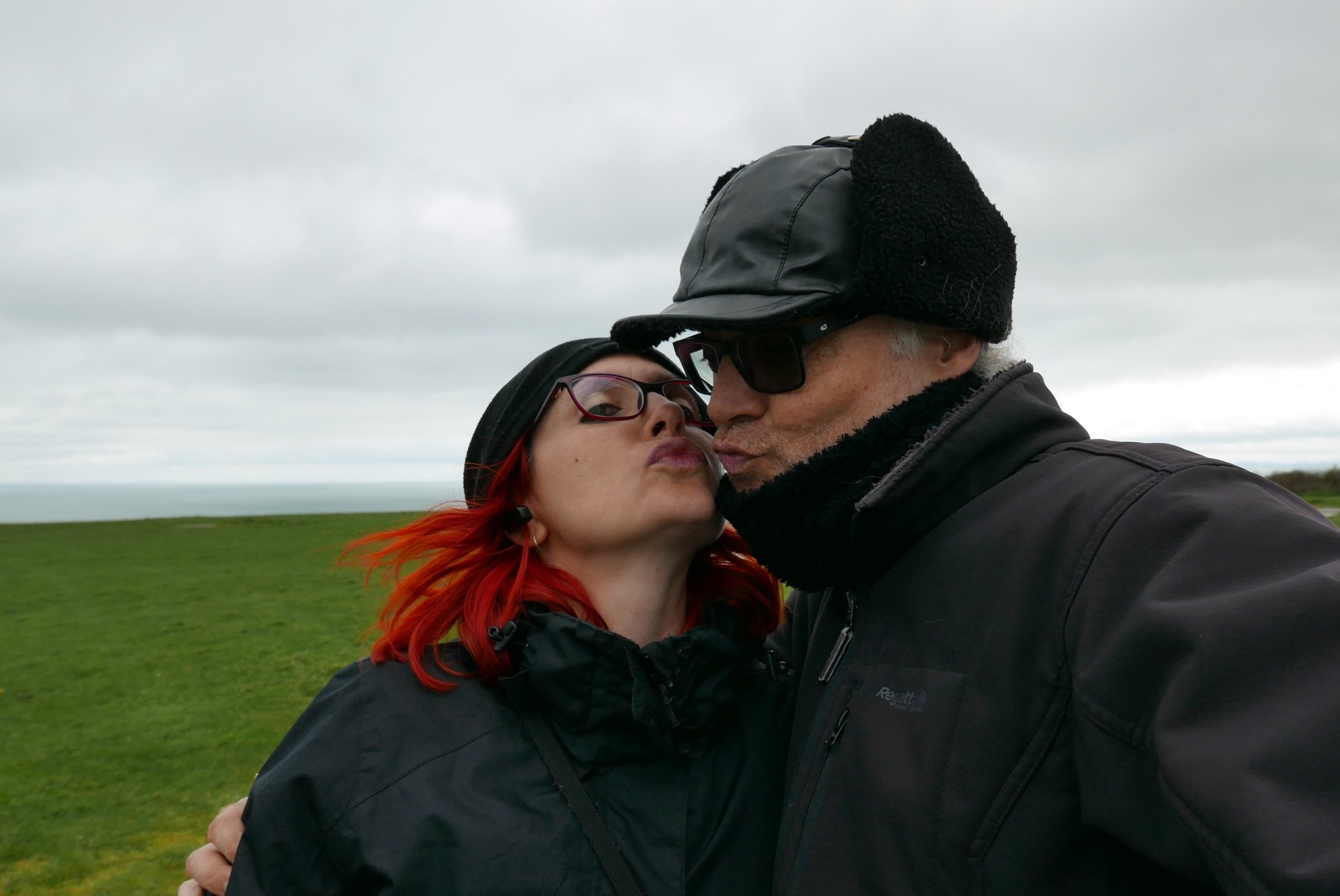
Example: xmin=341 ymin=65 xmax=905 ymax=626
xmin=226 ymin=337 xmax=799 ymax=896
xmin=175 ymin=115 xmax=1340 ymax=896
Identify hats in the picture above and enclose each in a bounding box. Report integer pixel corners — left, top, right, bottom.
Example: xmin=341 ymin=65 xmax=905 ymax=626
xmin=464 ymin=337 xmax=688 ymax=510
xmin=610 ymin=113 xmax=1017 ymax=351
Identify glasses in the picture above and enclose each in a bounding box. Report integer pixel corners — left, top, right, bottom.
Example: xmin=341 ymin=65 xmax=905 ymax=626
xmin=510 ymin=372 xmax=714 ymax=497
xmin=673 ymin=308 xmax=874 ymax=394
